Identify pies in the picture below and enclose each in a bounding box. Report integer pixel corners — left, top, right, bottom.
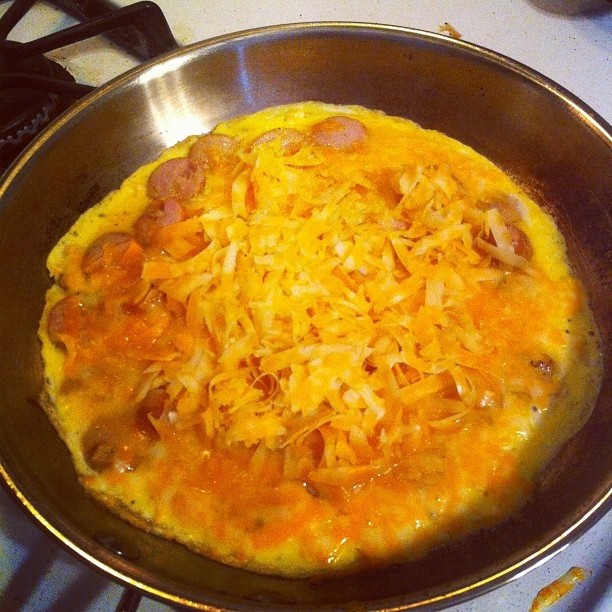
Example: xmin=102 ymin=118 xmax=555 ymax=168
xmin=40 ymin=97 xmax=604 ymax=580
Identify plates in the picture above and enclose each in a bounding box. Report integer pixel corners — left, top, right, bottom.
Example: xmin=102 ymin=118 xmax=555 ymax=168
xmin=0 ymin=21 xmax=612 ymax=611
